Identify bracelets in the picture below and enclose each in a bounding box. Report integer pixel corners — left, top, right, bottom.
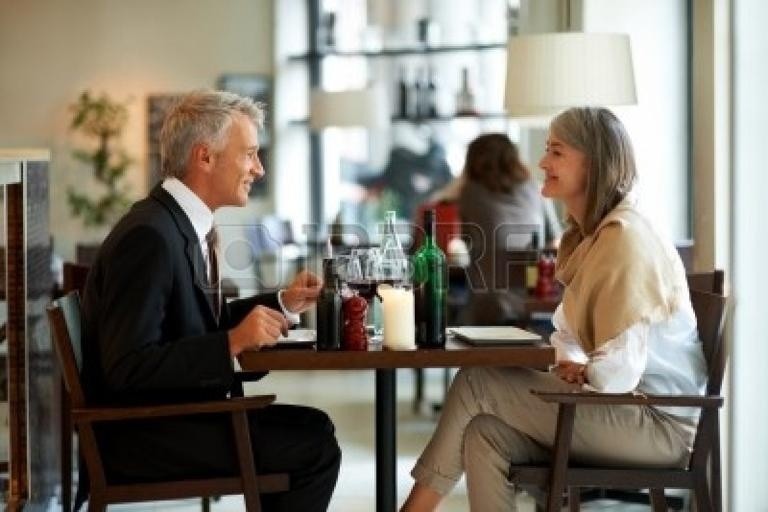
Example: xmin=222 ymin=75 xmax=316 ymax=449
xmin=577 ymin=362 xmax=587 ymax=377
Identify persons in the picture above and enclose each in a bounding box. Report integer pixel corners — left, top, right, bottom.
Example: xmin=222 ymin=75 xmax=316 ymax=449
xmin=399 ymin=106 xmax=709 ymax=510
xmin=81 ymin=86 xmax=345 ymax=511
xmin=345 ymin=136 xmax=463 ymax=242
xmin=446 ymin=129 xmax=557 ymax=330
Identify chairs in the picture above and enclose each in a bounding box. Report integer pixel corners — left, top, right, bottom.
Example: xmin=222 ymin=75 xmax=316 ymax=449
xmin=508 ymin=270 xmax=728 ymax=512
xmin=46 ymin=288 xmax=289 ymax=512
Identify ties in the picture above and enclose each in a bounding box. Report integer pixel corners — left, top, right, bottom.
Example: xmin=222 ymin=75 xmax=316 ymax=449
xmin=206 ymin=226 xmax=221 ymax=317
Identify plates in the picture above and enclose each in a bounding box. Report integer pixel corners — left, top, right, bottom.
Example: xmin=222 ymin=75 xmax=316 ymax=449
xmin=450 ymin=324 xmax=543 ymax=345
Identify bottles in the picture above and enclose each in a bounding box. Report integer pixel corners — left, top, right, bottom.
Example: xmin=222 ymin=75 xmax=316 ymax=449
xmin=315 ymin=208 xmax=449 ymax=350
